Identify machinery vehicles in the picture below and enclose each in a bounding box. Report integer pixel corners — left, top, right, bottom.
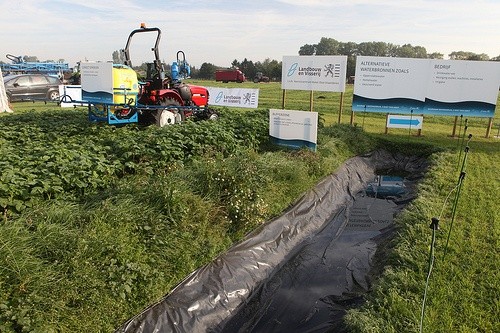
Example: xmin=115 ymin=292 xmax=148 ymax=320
xmin=252 ymin=71 xmax=269 ymax=84
xmin=123 ymin=20 xmax=221 ymax=128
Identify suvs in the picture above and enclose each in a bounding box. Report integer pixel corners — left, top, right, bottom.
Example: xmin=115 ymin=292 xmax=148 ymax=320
xmin=2 ymin=74 xmax=64 ymax=103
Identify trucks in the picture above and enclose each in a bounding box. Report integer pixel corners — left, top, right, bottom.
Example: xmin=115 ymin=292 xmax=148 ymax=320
xmin=214 ymin=67 xmax=245 ymax=85
